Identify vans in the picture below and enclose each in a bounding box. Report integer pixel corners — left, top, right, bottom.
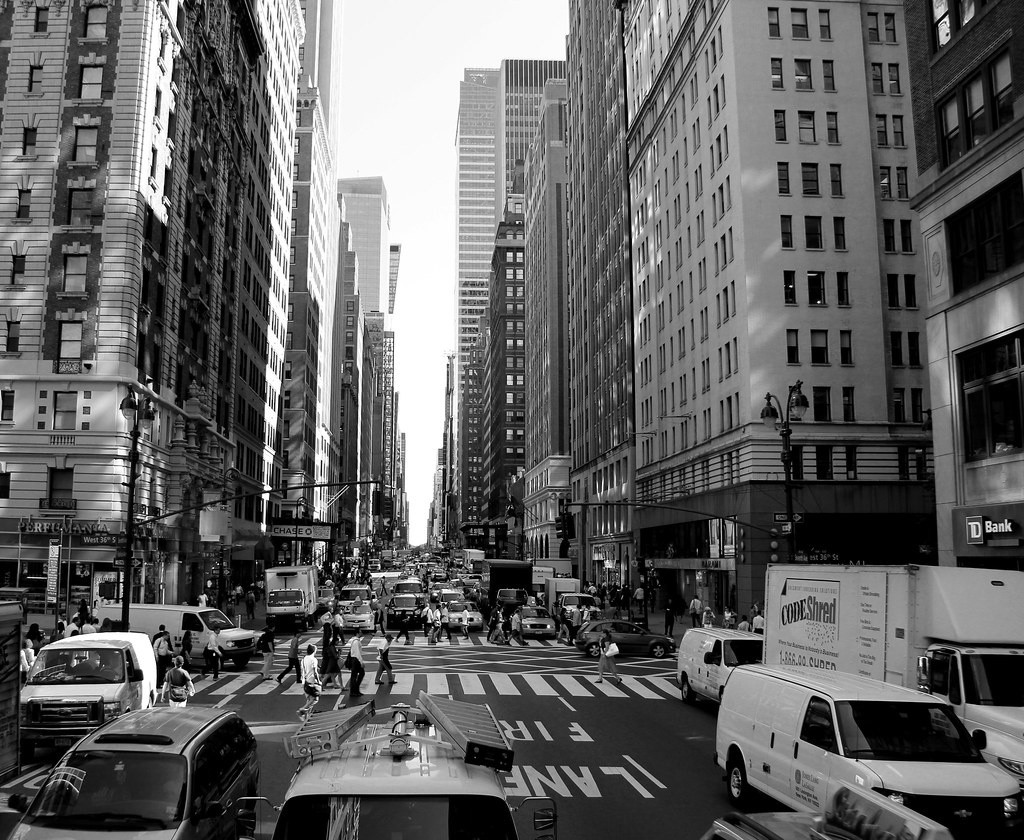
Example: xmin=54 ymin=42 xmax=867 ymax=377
xmin=715 ymin=662 xmax=1024 ymax=840
xmin=233 ymin=687 xmax=561 ymax=840
xmin=676 ymin=626 xmax=764 ymax=705
xmin=94 ymin=602 xmax=256 ymax=673
xmin=17 ymin=631 xmax=159 ymax=754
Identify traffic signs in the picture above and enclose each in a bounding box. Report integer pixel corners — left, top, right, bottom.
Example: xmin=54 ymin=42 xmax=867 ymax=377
xmin=767 ymin=521 xmax=792 ymax=537
xmin=114 ymin=557 xmax=144 ymax=569
xmin=772 ymin=512 xmax=806 ymax=524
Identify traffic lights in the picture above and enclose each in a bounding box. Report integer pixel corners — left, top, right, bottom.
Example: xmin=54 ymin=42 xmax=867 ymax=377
xmin=636 ymin=556 xmax=646 ymax=573
xmin=555 ymin=512 xmax=567 ymax=540
xmin=381 ymin=495 xmax=393 ymax=518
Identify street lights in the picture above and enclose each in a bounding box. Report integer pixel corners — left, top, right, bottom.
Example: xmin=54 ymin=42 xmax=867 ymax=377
xmin=118 ymin=383 xmax=155 ymax=631
xmin=759 ymin=379 xmax=811 ymax=563
xmin=218 ymin=467 xmax=243 ymax=613
xmin=295 ymin=496 xmax=310 ymax=566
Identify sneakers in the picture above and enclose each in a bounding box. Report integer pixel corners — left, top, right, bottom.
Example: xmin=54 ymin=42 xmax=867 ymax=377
xmin=276 ymin=678 xmax=283 ymax=684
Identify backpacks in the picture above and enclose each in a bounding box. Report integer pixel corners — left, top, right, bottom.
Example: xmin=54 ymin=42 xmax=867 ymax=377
xmin=257 ymin=634 xmax=267 ymax=652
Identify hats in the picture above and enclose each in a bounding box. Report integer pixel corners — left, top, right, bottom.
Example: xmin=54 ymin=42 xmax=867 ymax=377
xmin=22 ymin=640 xmax=33 ymax=648
xmin=386 ymin=634 xmax=393 ymax=640
xmin=173 ymin=656 xmax=184 ymax=666
xmin=704 ymin=607 xmax=711 ymax=611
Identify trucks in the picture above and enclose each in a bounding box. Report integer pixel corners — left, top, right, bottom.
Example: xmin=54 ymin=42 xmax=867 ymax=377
xmin=264 ymin=565 xmax=321 ymax=633
xmin=544 ymin=577 xmax=603 ymax=633
xmin=761 ymin=564 xmax=1024 ymax=795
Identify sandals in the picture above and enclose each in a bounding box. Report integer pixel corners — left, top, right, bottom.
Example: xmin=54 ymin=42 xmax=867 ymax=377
xmin=261 ymin=674 xmax=273 ymax=680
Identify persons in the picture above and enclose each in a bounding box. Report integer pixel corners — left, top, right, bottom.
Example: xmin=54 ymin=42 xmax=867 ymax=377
xmin=594 ymin=629 xmax=622 ymax=685
xmin=584 ymin=580 xmax=644 ymax=615
xmin=180 ymin=630 xmax=193 ymax=675
xmin=275 ymin=631 xmax=302 ymax=683
xmin=319 ymin=608 xmax=365 ymax=696
xmin=152 ymin=624 xmax=175 ymax=688
xmin=161 ymin=656 xmax=195 ymax=707
xmin=375 ymin=635 xmax=397 ymax=684
xmin=371 ymin=604 xmax=386 ymax=637
xmin=199 ymin=626 xmax=223 ymax=681
xmin=296 ymin=644 xmax=322 ymax=722
xmin=259 ymin=624 xmax=275 ymax=680
xmin=487 ymin=601 xmax=530 ymax=646
xmin=21 ymin=599 xmax=111 ymax=683
xmin=688 ymin=595 xmax=764 ymax=635
xmin=317 ymin=558 xmax=389 ymax=596
xmin=395 ymin=603 xmax=471 ymax=645
xmin=557 ymin=605 xmax=591 ymax=645
xmin=196 ymin=577 xmax=264 ymax=620
xmin=664 ymin=598 xmax=678 ymax=637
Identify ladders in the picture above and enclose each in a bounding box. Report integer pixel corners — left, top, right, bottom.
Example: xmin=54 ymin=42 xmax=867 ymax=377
xmin=416 ymin=689 xmax=515 ymax=772
xmin=291 ymin=699 xmax=376 ymax=758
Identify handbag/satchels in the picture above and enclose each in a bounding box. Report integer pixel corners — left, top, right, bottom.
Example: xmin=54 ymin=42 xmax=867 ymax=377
xmin=344 ymin=653 xmax=352 ymax=668
xmin=688 ymin=607 xmax=697 ymax=614
xmin=247 ymin=598 xmax=256 ymax=607
xmin=169 ymin=687 xmax=187 ymax=702
xmin=732 ymin=611 xmax=738 ymax=622
xmin=336 ymin=657 xmax=344 ymax=672
xmin=601 ymin=643 xmax=621 ymax=658
xmin=433 ymin=620 xmax=441 ymax=627
xmin=304 ymin=682 xmax=322 ymax=697
xmin=419 ymin=615 xmax=429 ymax=623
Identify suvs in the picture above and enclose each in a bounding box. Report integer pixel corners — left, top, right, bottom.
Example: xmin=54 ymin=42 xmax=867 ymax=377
xmin=517 ymin=596 xmax=557 ymax=640
xmin=3 ymin=704 xmax=259 ymax=840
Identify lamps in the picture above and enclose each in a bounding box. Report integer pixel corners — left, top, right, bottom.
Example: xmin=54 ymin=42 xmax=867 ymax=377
xmin=84 ymin=363 xmax=93 ymax=370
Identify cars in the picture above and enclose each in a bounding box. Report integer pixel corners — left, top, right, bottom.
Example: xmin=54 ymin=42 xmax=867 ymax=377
xmin=572 ymin=618 xmax=676 ymax=659
xmin=700 ymin=776 xmax=953 ymax=840
xmin=445 ymin=598 xmax=484 ymax=632
xmin=385 ymin=594 xmax=428 ymax=630
xmin=316 ymin=548 xmax=557 ymax=634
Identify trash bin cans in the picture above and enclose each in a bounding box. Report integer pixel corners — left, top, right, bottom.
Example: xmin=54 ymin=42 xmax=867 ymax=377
xmin=632 ymin=616 xmax=646 ymax=632
xmin=226 ymin=599 xmax=235 ymax=617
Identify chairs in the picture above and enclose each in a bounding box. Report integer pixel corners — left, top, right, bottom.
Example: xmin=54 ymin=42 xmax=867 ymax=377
xmin=59 ymin=655 xmax=71 ymax=666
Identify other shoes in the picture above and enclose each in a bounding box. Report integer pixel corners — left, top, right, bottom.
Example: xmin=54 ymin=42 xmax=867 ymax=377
xmin=295 ymin=709 xmax=307 ymax=722
xmin=487 ymin=639 xmax=529 ymax=647
xmin=557 ymin=639 xmax=574 ymax=646
xmin=389 ymin=681 xmax=397 ymax=685
xmin=615 ymin=678 xmax=622 ymax=685
xmin=375 ymin=682 xmax=384 ymax=684
xmin=595 ymin=680 xmax=603 ymax=683
xmin=371 ymin=633 xmax=375 ymax=636
xmin=380 ymin=635 xmax=385 ymax=637
xmin=431 ymin=632 xmax=469 ymax=642
